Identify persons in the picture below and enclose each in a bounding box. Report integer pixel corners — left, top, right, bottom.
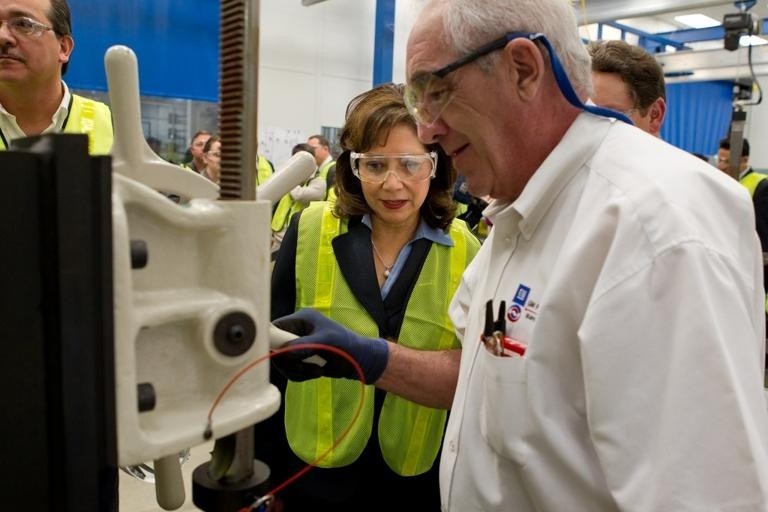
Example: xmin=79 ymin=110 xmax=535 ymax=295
xmin=718 ymin=135 xmax=768 ymax=389
xmin=270 ymin=83 xmax=483 ymax=512
xmin=0 ymin=0 xmax=115 ymax=156
xmin=270 ymin=0 xmax=767 ymax=512
xmin=257 ymin=134 xmax=341 ymax=242
xmin=587 ymin=40 xmax=667 ymax=141
xmin=144 ymin=131 xmax=221 ymax=185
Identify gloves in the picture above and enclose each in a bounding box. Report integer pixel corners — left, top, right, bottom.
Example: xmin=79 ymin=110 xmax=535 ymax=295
xmin=267 ymin=306 xmax=391 ymax=387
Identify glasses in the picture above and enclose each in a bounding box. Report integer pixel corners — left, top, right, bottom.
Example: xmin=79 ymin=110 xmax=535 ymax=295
xmin=399 ymin=31 xmax=538 ymax=129
xmin=1 ymin=16 xmax=54 ymax=38
xmin=347 ymin=149 xmax=439 ymax=184
